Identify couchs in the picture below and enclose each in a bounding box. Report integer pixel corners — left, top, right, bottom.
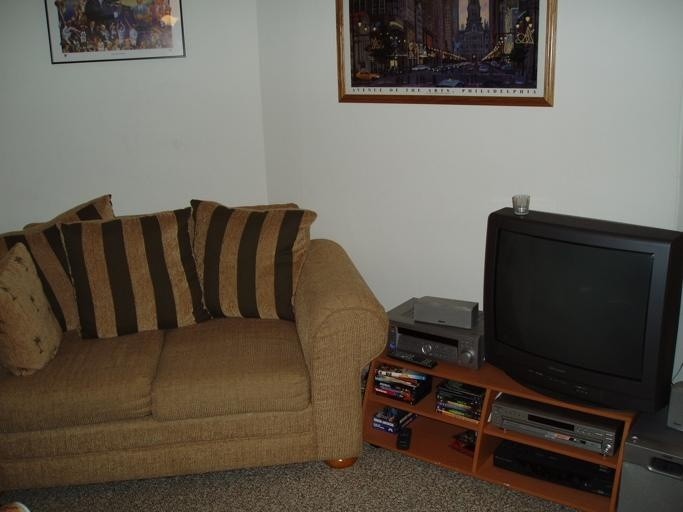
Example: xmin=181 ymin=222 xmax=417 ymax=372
xmin=0 ymin=193 xmax=388 ymax=491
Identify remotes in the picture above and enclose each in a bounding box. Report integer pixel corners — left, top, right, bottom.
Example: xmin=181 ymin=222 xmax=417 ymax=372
xmin=386 ymin=349 xmax=437 ymax=369
xmin=396 ymin=427 xmax=410 ymax=449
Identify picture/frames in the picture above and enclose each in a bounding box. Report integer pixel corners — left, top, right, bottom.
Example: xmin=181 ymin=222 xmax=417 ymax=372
xmin=335 ymin=1 xmax=558 ymax=107
xmin=45 ymin=0 xmax=185 ymax=63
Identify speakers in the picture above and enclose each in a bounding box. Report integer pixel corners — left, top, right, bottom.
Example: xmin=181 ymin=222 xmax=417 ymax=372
xmin=667 ymin=381 xmax=683 ymax=431
xmin=413 ymin=294 xmax=478 ymax=328
xmin=616 ymin=403 xmax=683 ymax=511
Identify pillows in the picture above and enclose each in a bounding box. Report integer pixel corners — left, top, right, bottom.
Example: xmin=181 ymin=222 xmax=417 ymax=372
xmin=191 ymin=199 xmax=317 ymax=320
xmin=2 ymin=195 xmax=118 ymax=331
xmin=0 ymin=243 xmax=63 ymax=379
xmin=56 ymin=206 xmax=213 ymax=339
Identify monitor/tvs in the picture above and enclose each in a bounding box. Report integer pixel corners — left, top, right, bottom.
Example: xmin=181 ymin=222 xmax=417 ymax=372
xmin=482 ymin=208 xmax=681 ymax=414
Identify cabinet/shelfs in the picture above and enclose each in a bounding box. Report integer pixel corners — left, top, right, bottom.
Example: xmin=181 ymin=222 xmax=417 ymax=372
xmin=362 ymin=348 xmax=637 ymax=512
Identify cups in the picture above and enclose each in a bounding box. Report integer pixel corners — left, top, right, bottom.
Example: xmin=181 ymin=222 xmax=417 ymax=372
xmin=512 ymin=195 xmax=531 ymax=216
